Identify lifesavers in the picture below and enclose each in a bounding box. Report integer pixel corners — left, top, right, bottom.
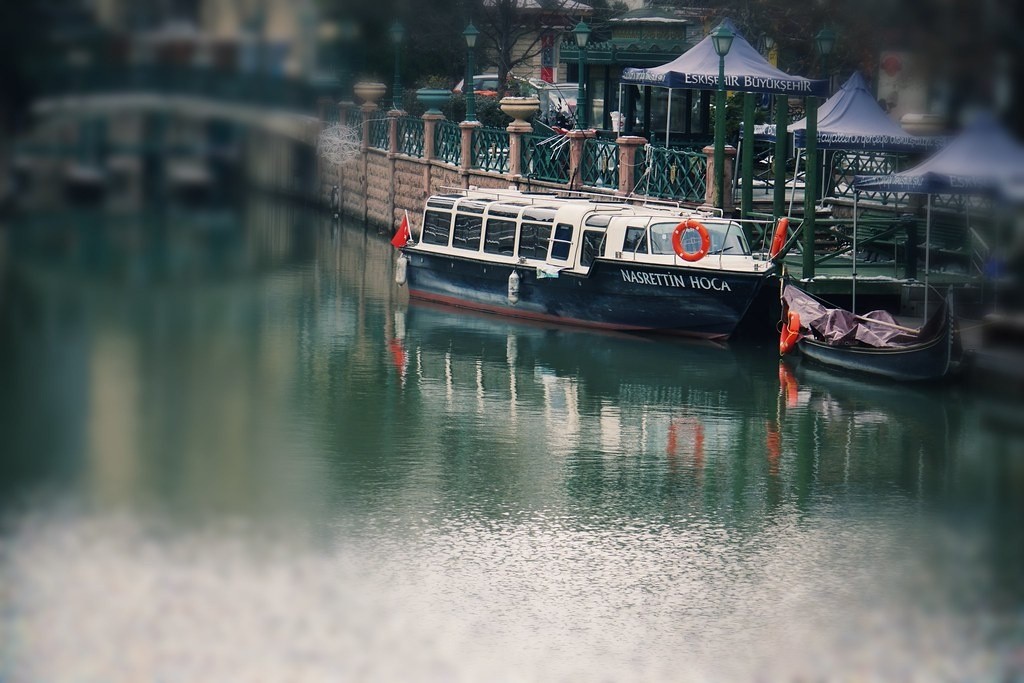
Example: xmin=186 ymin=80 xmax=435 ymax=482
xmin=669 ymin=221 xmax=708 ymax=262
xmin=777 ymin=364 xmax=798 ymax=407
xmin=779 ymin=312 xmax=800 ymax=354
xmin=772 ymin=219 xmax=786 ymax=256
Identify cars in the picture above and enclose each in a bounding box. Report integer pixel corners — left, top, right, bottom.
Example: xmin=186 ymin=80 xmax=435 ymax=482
xmin=451 ymin=72 xmax=587 ymax=120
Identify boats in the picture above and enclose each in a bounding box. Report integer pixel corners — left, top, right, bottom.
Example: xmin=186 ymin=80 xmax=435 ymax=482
xmin=777 ymin=264 xmax=963 ymax=391
xmin=390 ymin=184 xmax=789 ymax=343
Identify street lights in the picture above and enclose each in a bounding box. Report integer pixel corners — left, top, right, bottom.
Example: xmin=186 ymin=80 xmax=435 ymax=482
xmin=710 ymin=23 xmax=735 ymax=217
xmin=462 ymin=17 xmax=480 ymax=166
xmin=570 ymin=15 xmax=592 ymax=129
xmin=816 ymin=22 xmax=836 ymax=107
xmin=388 ymin=17 xmax=405 ymax=111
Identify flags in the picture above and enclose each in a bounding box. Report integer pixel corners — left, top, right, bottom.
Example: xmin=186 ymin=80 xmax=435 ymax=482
xmin=390 ymin=214 xmax=409 ymax=249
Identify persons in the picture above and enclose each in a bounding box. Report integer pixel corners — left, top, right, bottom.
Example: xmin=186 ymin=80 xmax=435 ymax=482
xmin=503 ymin=71 xmax=521 ymax=98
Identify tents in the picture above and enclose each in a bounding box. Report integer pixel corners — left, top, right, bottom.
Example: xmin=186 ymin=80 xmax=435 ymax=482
xmin=849 ymin=102 xmax=1024 ymax=344
xmin=731 ymin=62 xmax=962 ymax=258
xmin=617 ymin=15 xmax=823 ymax=213
